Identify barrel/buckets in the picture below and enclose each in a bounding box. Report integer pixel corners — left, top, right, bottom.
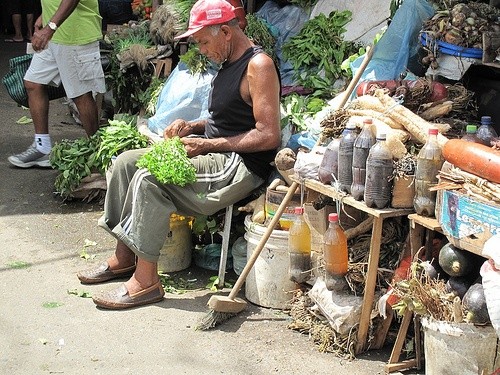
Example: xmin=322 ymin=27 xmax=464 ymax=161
xmin=242 ymin=214 xmax=294 ymax=311
xmin=420 ymin=316 xmax=500 ymax=375
xmin=157 ymin=213 xmax=191 ymax=274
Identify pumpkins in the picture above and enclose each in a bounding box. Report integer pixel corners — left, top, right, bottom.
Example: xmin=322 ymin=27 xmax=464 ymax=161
xmin=422 ymin=231 xmax=492 ymax=325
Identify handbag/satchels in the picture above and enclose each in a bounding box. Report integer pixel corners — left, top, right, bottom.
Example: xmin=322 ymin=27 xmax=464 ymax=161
xmin=3 ymin=55 xmax=68 ymax=107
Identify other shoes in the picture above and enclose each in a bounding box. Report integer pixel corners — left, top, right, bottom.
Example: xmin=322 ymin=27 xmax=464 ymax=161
xmin=93 ymin=275 xmax=165 ymax=310
xmin=4 ymin=35 xmax=24 ymax=42
xmin=77 ymin=262 xmax=137 ymax=283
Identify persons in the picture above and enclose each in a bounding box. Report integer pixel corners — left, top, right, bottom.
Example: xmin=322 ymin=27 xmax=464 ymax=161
xmin=227 ymin=0 xmax=246 ymax=27
xmin=76 ymin=0 xmax=281 ymax=308
xmin=8 ymin=0 xmax=107 ymax=170
xmin=5 ymin=0 xmax=36 ymax=43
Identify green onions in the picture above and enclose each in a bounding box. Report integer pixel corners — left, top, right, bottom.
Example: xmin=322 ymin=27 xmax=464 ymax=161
xmin=111 ymin=0 xmax=196 ymax=67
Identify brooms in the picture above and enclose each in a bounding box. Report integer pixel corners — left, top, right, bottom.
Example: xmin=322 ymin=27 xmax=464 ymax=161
xmin=194 ymin=43 xmax=377 ymax=332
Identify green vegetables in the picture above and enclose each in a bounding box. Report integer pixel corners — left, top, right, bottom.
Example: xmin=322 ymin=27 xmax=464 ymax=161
xmin=49 ymin=67 xmax=164 ymax=201
xmin=242 ymin=13 xmax=278 ymax=64
xmin=135 ymin=134 xmax=197 ymax=188
xmin=280 ymin=0 xmax=366 ymax=133
xmin=180 ymin=43 xmax=211 ymax=77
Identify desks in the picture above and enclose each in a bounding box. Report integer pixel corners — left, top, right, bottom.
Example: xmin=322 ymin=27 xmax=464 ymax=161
xmin=385 ymin=213 xmax=500 ymax=375
xmin=290 ymin=175 xmax=413 ymax=355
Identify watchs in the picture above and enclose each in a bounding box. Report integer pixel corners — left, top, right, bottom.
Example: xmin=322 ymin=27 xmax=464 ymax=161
xmin=47 ymin=21 xmax=58 ymax=31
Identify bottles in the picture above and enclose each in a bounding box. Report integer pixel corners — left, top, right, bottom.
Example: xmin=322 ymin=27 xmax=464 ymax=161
xmin=287 ymin=206 xmax=312 ymax=284
xmin=477 ymin=116 xmax=498 ymax=146
xmin=460 ymin=125 xmax=484 ymax=144
xmin=364 ymin=133 xmax=393 ymax=208
xmin=317 ymin=137 xmax=341 ymax=184
xmin=351 ymin=118 xmax=377 ymax=199
xmin=337 ymin=123 xmax=360 ymax=194
xmin=324 ymin=213 xmax=349 ymax=291
xmin=413 ymin=128 xmax=443 ymax=217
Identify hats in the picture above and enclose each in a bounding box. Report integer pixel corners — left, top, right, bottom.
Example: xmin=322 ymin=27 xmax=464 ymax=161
xmin=174 ymin=0 xmax=237 ymax=39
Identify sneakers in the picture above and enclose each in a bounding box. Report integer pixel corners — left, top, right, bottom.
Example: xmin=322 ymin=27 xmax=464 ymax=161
xmin=8 ymin=141 xmax=52 ymax=167
